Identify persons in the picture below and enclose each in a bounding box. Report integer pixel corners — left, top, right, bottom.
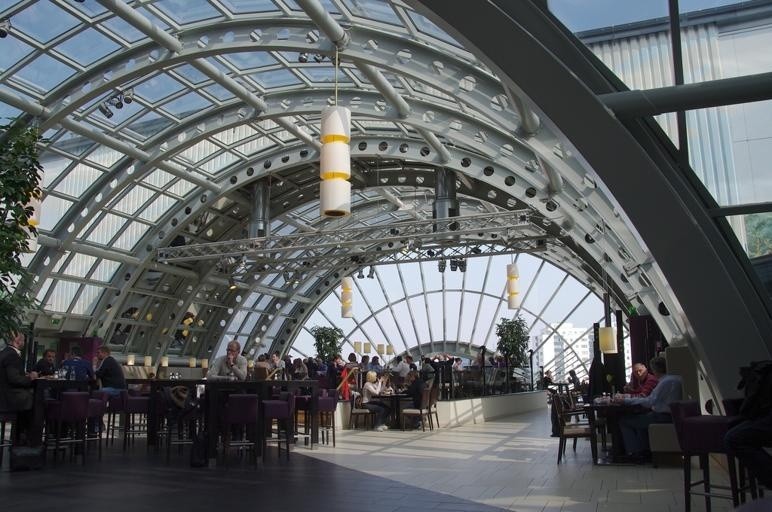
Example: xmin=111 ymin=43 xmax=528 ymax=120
xmin=141 ymin=372 xmax=155 ymax=392
xmin=723 ymin=360 xmax=772 ymax=491
xmin=242 ymin=351 xmax=463 ymax=431
xmin=56 ymin=346 xmax=94 ymax=438
xmin=623 ymin=362 xmax=658 ymax=398
xmin=30 ymin=348 xmax=55 ymax=376
xmin=569 ymin=370 xmax=582 ymax=391
xmin=93 ymin=344 xmax=125 ymax=431
xmin=0 ymin=330 xmax=45 ymax=450
xmin=613 ymin=356 xmax=682 ymax=463
xmin=206 ymin=340 xmax=248 ymax=440
xmin=543 ymin=370 xmax=558 ymax=394
xmin=492 ymin=356 xmax=504 ymax=368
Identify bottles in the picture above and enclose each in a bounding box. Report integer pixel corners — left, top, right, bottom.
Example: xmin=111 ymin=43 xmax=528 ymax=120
xmin=53 ymin=368 xmax=75 ymax=380
xmin=170 ymin=372 xmax=181 ymax=379
xmin=384 ymin=388 xmax=401 ymax=394
xmin=598 ymin=393 xmax=639 ymax=404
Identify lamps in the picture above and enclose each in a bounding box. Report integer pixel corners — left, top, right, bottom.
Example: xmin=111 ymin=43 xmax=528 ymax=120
xmin=14 ymin=120 xmax=43 ymax=254
xmin=228 ymin=277 xmax=237 ymax=290
xmin=320 ymin=44 xmax=350 ymax=218
xmin=598 ymin=222 xmax=618 ymax=354
xmin=506 ymin=253 xmax=520 ymax=310
xmin=354 ymin=342 xmax=394 ymax=373
xmin=98 ymin=89 xmax=135 ymax=119
xmin=356 ymin=266 xmax=374 ymax=279
xmin=283 ymin=271 xmax=301 ymax=284
xmin=438 ymin=258 xmax=467 ymax=272
xmin=0 ymin=18 xmax=12 ymax=37
xmin=299 ymin=50 xmax=340 ymax=68
xmin=341 ymin=269 xmax=353 ymax=319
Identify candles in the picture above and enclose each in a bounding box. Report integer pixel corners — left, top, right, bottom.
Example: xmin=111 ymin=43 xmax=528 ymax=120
xmin=127 ymin=355 xmax=208 ymax=368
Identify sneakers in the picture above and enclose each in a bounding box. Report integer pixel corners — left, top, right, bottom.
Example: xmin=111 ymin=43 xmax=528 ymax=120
xmin=373 ymin=423 xmax=389 ymax=432
